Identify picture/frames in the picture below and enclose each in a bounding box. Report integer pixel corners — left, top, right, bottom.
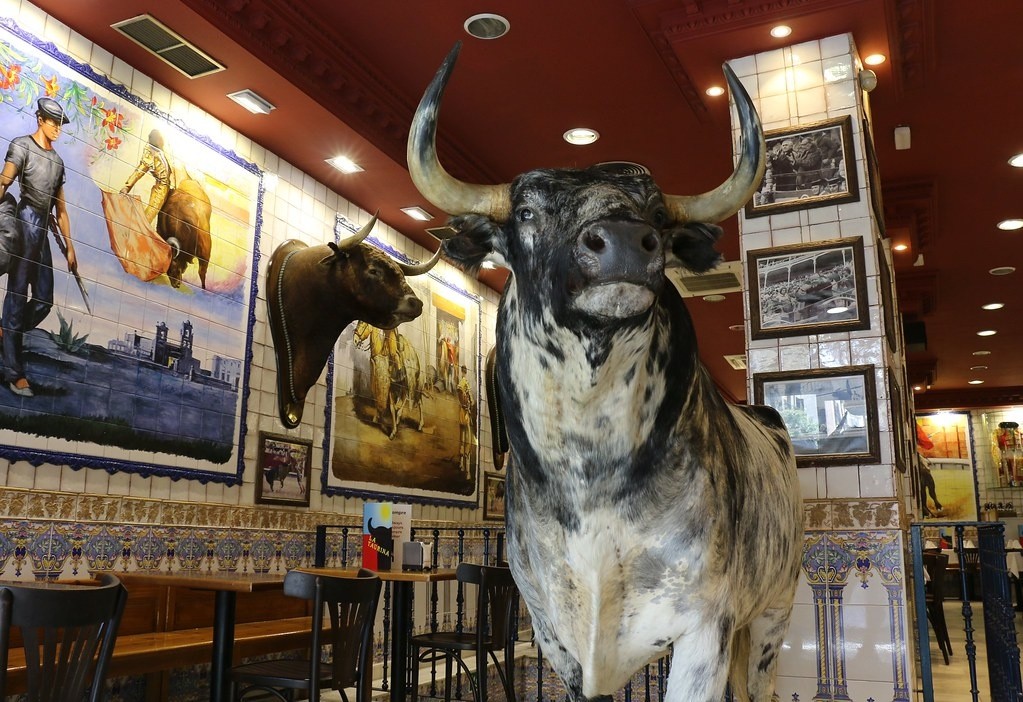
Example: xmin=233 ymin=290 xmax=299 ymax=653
xmin=250 ymin=430 xmax=312 ymax=507
xmin=744 ymin=235 xmax=873 ymax=341
xmin=483 ymin=471 xmax=507 ymax=522
xmin=751 ymin=364 xmax=880 ymax=470
xmin=740 ymin=115 xmax=860 ymax=220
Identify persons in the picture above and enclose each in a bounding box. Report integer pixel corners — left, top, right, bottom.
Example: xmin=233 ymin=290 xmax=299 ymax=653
xmin=264 ymin=439 xmax=307 ymax=493
xmin=754 ymin=130 xmax=848 ymax=206
xmin=758 ymin=251 xmax=854 ymax=322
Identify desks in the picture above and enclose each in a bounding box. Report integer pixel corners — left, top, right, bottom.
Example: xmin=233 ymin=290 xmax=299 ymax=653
xmin=302 ymin=563 xmax=459 ymax=702
xmin=88 ymin=569 xmax=287 ymax=702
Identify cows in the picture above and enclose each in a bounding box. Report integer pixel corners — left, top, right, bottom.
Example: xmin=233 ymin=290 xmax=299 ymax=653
xmin=276 ymin=212 xmax=447 ymax=402
xmin=407 ymin=41 xmax=806 ymax=696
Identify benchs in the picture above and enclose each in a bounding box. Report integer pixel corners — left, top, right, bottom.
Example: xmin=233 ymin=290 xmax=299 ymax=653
xmin=6 ymin=615 xmax=358 ymax=701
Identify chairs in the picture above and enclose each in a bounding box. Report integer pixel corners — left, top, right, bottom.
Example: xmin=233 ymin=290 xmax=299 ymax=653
xmin=923 ymin=551 xmax=954 ymax=666
xmin=0 ymin=574 xmax=129 ymax=702
xmin=409 ymin=563 xmax=519 ymax=702
xmin=231 ymin=570 xmax=382 ymax=701
